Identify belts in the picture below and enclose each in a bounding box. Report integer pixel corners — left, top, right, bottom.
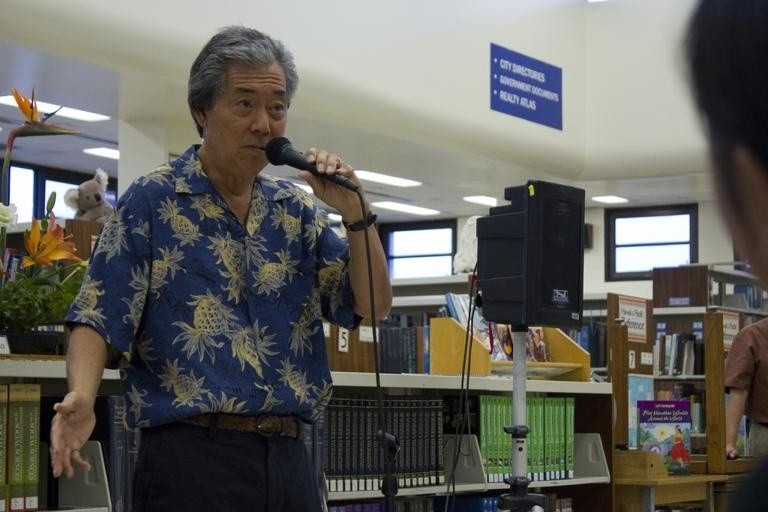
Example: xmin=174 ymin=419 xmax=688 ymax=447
xmin=186 ymin=413 xmax=306 ymax=441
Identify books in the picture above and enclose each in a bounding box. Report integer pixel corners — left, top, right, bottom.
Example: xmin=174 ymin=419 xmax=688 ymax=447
xmin=378 ymin=292 xmax=550 ymax=375
xmin=323 ymin=394 xmax=575 ymax=512
xmin=1 ymin=245 xmax=29 ymax=284
xmin=636 ymin=397 xmax=692 ymax=473
xmin=711 ymin=281 xmax=767 ymax=347
xmin=646 ymin=329 xmax=705 ymax=376
xmin=0 ymin=380 xmax=141 ymax=509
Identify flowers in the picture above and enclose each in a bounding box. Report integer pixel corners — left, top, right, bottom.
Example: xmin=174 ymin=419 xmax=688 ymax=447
xmin=0 ymin=86 xmax=93 ymax=333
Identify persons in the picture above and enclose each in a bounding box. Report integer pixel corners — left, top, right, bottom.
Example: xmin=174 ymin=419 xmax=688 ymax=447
xmin=673 ymin=423 xmax=682 ymax=445
xmin=722 ymin=317 xmax=768 ymax=460
xmin=667 ymin=428 xmax=690 ymax=470
xmin=49 ymin=25 xmax=395 ymax=511
xmin=681 ymin=0 xmax=768 ymax=509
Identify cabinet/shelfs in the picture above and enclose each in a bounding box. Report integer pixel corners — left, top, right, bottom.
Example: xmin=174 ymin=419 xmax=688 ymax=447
xmin=1 ymin=360 xmax=615 ymax=512
xmin=653 ymin=268 xmax=768 ymax=509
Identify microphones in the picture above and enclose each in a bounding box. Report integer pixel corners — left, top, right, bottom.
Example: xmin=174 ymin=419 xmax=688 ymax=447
xmin=264 ymin=135 xmax=360 ymax=194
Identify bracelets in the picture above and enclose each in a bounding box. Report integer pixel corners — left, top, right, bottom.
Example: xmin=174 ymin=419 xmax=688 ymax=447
xmin=343 ymin=208 xmax=377 ymax=234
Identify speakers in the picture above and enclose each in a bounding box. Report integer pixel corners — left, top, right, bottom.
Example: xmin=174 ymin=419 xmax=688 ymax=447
xmin=475 ymin=179 xmax=585 ymax=330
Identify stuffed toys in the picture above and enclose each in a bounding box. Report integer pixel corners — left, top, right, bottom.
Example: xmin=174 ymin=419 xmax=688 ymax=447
xmin=63 ymin=165 xmax=116 ymax=225
